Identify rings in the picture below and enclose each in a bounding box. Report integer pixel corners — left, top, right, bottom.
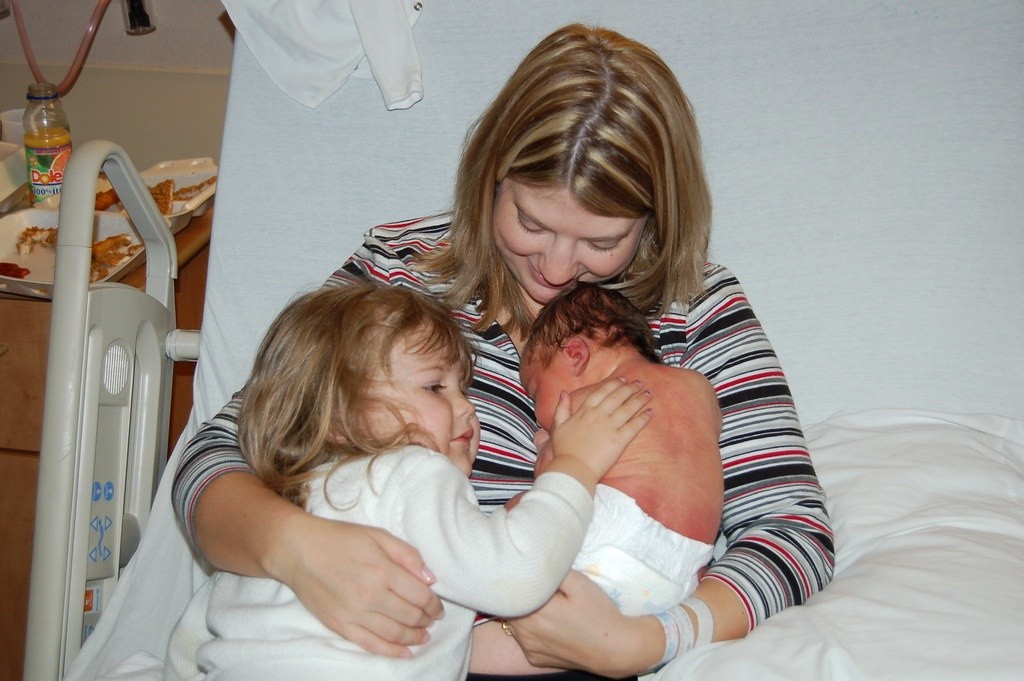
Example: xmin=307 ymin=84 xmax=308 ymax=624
xmin=502 ymin=621 xmax=512 ymax=636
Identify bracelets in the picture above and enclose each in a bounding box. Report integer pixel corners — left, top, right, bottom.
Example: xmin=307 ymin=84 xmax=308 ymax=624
xmin=656 ymin=613 xmax=678 ymax=668
xmin=682 ymin=596 xmax=714 ymax=649
xmin=668 ymin=605 xmax=694 ymax=657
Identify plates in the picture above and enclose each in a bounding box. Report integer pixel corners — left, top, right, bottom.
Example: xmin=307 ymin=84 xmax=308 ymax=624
xmin=0 ymin=200 xmax=150 ymax=299
xmin=85 ymin=155 xmax=219 ymax=236
xmin=0 ymin=141 xmax=35 ymax=215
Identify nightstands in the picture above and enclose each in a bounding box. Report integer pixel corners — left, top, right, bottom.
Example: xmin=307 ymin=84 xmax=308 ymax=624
xmin=0 ymin=205 xmax=218 ymax=681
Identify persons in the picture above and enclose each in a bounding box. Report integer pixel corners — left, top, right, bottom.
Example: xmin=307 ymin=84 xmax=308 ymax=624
xmin=171 ymin=23 xmax=833 ymax=681
xmin=198 ymin=284 xmax=653 ymax=681
xmin=504 ymin=286 xmax=725 ymax=618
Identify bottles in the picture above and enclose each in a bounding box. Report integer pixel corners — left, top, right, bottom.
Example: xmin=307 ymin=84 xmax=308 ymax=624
xmin=0 ymin=106 xmax=38 ymax=146
xmin=22 ymin=84 xmax=73 ymax=207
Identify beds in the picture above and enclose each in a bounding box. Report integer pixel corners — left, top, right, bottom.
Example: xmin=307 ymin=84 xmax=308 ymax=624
xmin=23 ymin=0 xmax=1024 ymax=681
